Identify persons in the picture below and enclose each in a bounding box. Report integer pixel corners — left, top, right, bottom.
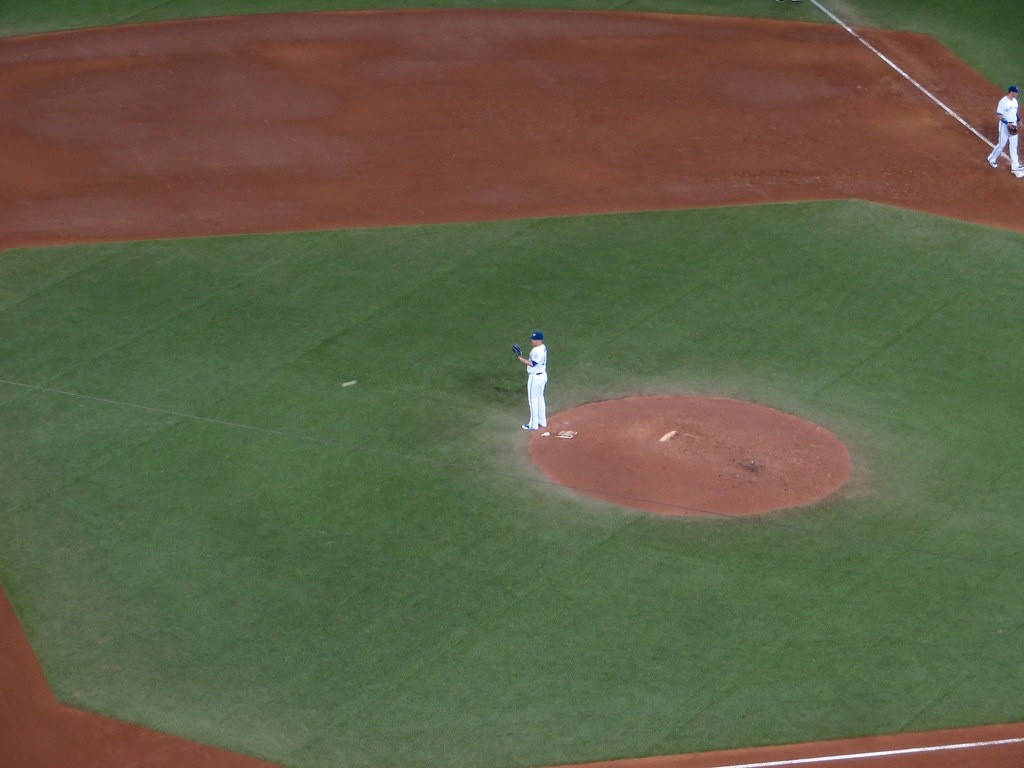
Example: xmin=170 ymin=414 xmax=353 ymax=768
xmin=987 ymin=86 xmax=1024 ymax=171
xmin=512 ymin=332 xmax=548 ymax=430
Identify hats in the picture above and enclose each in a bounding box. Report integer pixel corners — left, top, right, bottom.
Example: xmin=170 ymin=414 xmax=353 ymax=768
xmin=1009 ymin=86 xmax=1019 ymax=92
xmin=530 ymin=332 xmax=543 ymax=340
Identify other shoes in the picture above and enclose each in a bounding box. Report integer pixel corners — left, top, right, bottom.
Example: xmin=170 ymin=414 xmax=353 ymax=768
xmin=521 ymin=424 xmax=532 ymax=430
xmin=1012 ymin=166 xmax=1024 ymax=171
xmin=989 ymin=161 xmax=998 ymax=168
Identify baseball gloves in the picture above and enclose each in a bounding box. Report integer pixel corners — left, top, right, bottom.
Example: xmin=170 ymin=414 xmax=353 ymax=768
xmin=1007 ymin=124 xmax=1020 ymax=135
xmin=511 ymin=344 xmax=523 ymax=357
